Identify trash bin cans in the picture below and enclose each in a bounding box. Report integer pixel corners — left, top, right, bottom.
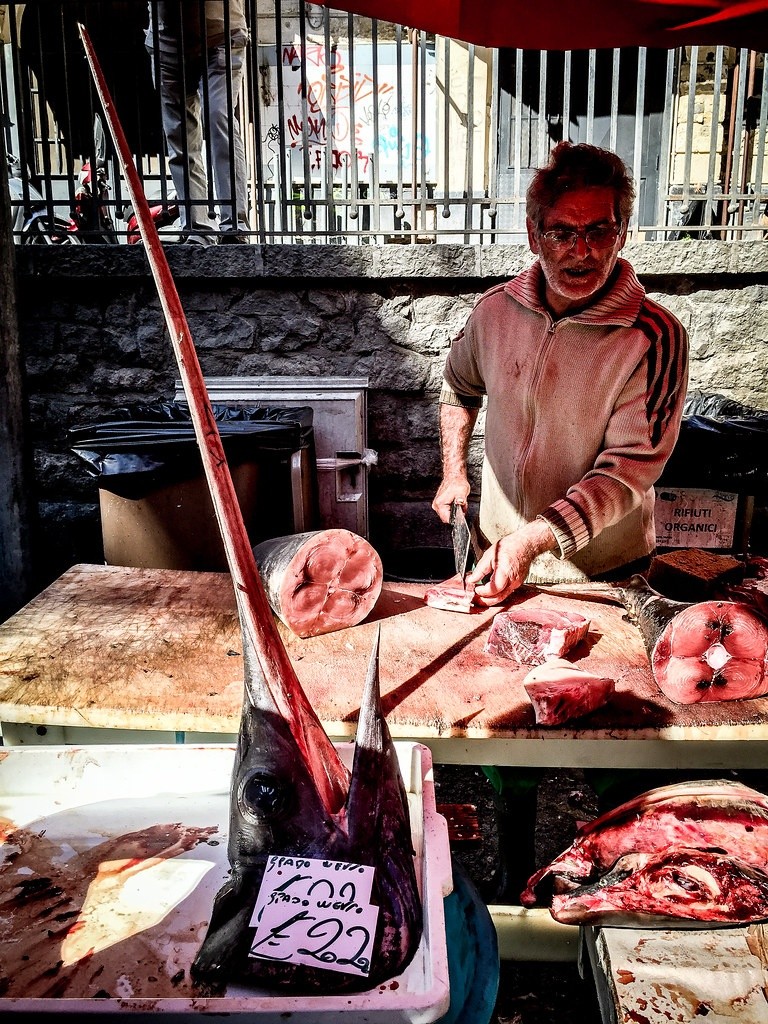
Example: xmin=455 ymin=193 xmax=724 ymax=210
xmin=65 ymin=404 xmax=316 ymax=574
xmin=654 ymin=394 xmax=767 ymax=561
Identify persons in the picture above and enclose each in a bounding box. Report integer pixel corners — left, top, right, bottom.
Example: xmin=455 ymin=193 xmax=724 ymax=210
xmin=431 ymin=144 xmax=690 ymax=901
xmin=144 ymin=0 xmax=251 ymax=244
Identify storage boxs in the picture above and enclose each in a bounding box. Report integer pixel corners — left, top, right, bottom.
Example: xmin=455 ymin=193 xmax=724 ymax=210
xmin=0 ymin=742 xmax=450 ymax=1023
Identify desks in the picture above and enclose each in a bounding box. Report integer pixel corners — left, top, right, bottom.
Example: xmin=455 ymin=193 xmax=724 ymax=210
xmin=0 ymin=560 xmax=768 ymax=771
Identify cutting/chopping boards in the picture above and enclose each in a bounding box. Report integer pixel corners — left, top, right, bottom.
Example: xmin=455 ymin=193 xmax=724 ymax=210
xmin=0 ymin=563 xmax=768 ymax=742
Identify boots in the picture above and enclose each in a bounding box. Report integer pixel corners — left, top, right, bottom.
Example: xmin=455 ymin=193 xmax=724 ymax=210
xmin=492 ymin=796 xmax=537 ymax=909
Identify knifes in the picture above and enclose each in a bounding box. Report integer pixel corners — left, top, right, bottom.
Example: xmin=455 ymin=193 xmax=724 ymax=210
xmin=447 ymin=500 xmax=471 ymax=579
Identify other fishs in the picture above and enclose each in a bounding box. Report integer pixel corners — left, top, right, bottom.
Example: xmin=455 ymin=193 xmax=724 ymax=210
xmin=249 ymin=526 xmax=768 ymax=730
xmin=519 ymin=778 xmax=768 ymax=931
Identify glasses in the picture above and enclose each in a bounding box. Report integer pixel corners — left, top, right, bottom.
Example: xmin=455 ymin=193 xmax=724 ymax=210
xmin=541 ymin=225 xmax=621 ymax=251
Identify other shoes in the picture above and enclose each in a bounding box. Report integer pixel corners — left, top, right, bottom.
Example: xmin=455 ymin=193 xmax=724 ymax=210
xmin=220 ymin=230 xmax=250 ymax=243
xmin=187 ymin=235 xmax=208 ymax=244
